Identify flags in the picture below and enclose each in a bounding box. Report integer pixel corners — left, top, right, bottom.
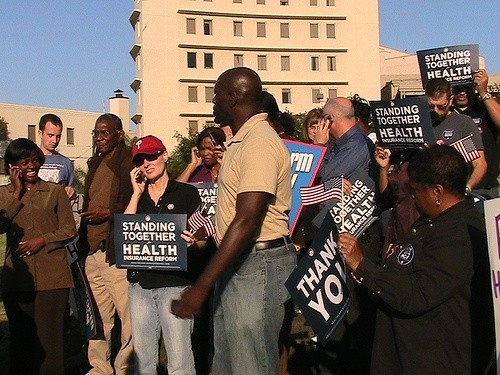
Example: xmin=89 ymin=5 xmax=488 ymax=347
xmin=453 ymin=138 xmax=481 ymax=163
xmin=187 ymin=203 xmax=216 ymax=238
xmin=299 ymin=177 xmax=342 ymax=205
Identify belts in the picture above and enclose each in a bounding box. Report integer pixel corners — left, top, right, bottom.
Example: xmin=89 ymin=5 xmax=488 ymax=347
xmin=247 ymin=235 xmax=292 ymax=252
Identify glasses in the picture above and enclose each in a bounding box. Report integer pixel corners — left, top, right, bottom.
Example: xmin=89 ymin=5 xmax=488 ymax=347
xmin=132 ymin=151 xmax=163 ymax=168
xmin=91 ymin=128 xmax=117 ymax=137
xmin=428 ymin=99 xmax=450 ymax=110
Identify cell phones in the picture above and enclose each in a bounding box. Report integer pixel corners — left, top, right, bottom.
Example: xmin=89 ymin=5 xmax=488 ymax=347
xmin=8 ymin=164 xmax=22 ymax=179
xmin=196 ymin=150 xmax=201 ymax=158
xmin=322 ymin=116 xmax=333 ymax=130
xmin=134 ymin=170 xmax=145 ymax=183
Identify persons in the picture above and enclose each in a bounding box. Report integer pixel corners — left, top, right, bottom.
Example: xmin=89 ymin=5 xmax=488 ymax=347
xmin=305 ymin=108 xmax=324 ymax=143
xmin=122 ymin=135 xmax=207 ymax=375
xmin=35 ymin=114 xmax=74 ymax=189
xmin=175 ymin=66 xmax=297 ymax=375
xmin=452 ymin=69 xmax=500 ymax=199
xmin=261 ymin=91 xmax=295 ymax=140
xmin=315 ymin=97 xmax=376 ymax=375
xmin=175 ymin=127 xmax=226 ymax=184
xmin=1 ymin=138 xmax=78 ymax=375
xmin=350 ymin=100 xmax=377 ymax=144
xmin=78 ymin=113 xmax=133 ymax=375
xmin=426 ymin=78 xmax=488 ymax=192
xmin=336 ymin=144 xmax=495 ymax=375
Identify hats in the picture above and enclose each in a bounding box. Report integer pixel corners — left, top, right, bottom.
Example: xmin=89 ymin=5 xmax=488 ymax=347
xmin=132 ymin=135 xmax=166 ymax=162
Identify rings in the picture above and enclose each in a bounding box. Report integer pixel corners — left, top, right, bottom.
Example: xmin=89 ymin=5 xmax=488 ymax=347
xmin=28 ymin=252 xmax=31 ymax=255
xmin=340 ymin=242 xmax=343 ymax=248
xmin=339 ymin=249 xmax=341 ymax=253
xmin=26 ymin=251 xmax=29 ymax=253
xmin=479 ymin=71 xmax=482 ymax=75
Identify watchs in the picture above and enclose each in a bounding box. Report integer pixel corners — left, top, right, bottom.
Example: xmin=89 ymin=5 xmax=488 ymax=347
xmin=481 ymin=92 xmax=491 ymax=104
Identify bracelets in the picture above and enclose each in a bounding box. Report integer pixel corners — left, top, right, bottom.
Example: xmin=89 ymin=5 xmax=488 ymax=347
xmin=465 ymin=184 xmax=471 ymax=192
